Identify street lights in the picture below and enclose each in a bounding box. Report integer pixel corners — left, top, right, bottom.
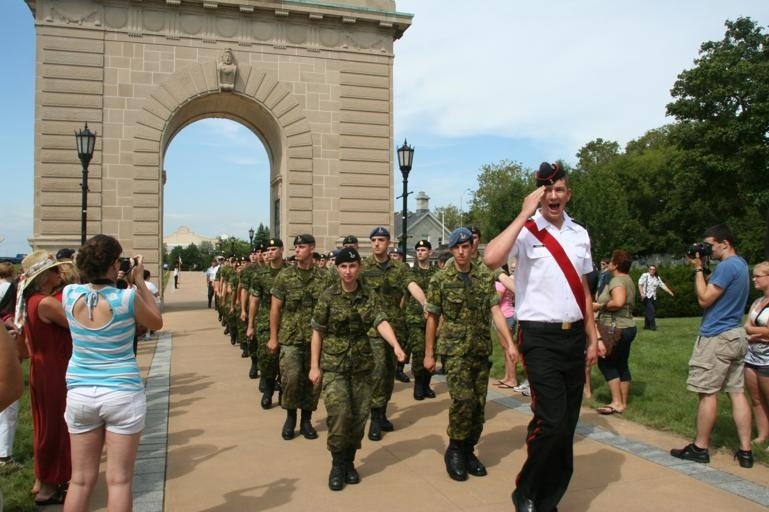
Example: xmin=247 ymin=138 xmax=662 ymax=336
xmin=219 ymin=238 xmax=223 ymax=256
xmin=441 ymin=211 xmax=446 ymax=244
xmin=396 ymin=138 xmax=416 ymax=265
xmin=460 ymin=188 xmax=471 ymax=227
xmin=73 ymin=120 xmax=99 ymax=245
xmin=248 ymin=227 xmax=255 ymax=250
xmin=230 ymin=235 xmax=236 ymax=257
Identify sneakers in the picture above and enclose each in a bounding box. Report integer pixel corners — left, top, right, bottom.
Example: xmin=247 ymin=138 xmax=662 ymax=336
xmin=514 ymin=382 xmax=529 ymax=392
xmin=734 ymin=448 xmax=754 ymax=468
xmin=671 ymin=444 xmax=710 ymax=463
xmin=522 ymin=387 xmax=531 ymax=396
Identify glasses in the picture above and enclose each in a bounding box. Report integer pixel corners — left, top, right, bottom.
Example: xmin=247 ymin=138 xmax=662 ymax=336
xmin=751 ymin=273 xmax=768 ymax=278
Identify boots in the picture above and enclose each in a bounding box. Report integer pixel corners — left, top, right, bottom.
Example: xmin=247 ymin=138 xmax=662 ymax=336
xmin=344 ymin=447 xmax=359 ymax=484
xmin=369 ymin=407 xmax=384 ymax=441
xmin=224 ymin=325 xmax=251 ymax=357
xmin=396 ymin=365 xmax=410 ymax=382
xmin=444 ymin=437 xmax=468 ymax=482
xmin=299 ymin=409 xmax=317 ymax=439
xmin=464 ymin=437 xmax=486 ymax=477
xmin=378 ymin=406 xmax=394 ymax=431
xmin=414 ymin=376 xmax=425 ymax=400
xmin=249 ymin=357 xmax=283 ymax=409
xmin=424 ymin=375 xmax=435 ymax=398
xmin=282 ymin=408 xmax=297 ymax=440
xmin=329 ymin=452 xmax=344 ymax=490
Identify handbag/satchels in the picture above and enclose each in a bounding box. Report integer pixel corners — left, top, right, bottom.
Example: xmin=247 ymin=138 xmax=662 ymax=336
xmin=594 ymin=306 xmax=623 ymax=359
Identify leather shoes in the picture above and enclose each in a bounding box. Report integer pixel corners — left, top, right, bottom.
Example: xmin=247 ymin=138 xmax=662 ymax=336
xmin=511 ymin=488 xmax=536 ymax=511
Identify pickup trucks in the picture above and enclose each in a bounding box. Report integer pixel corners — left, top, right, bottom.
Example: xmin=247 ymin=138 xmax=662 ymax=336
xmin=0 ymin=254 xmax=29 ymax=265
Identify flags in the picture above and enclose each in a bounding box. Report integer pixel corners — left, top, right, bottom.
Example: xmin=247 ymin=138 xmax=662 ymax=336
xmin=179 ymin=257 xmax=183 ymax=265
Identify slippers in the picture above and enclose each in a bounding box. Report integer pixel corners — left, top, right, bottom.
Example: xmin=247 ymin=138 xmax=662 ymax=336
xmin=35 ymin=491 xmax=66 ymax=505
xmin=491 ymin=380 xmax=505 ymax=385
xmin=498 ymin=383 xmax=514 ymax=388
xmin=597 ymin=404 xmax=622 ymax=414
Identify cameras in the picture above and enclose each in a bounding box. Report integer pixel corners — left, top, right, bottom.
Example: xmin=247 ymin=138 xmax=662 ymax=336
xmin=687 ymin=239 xmax=713 ymax=257
xmin=119 ymin=258 xmax=138 ymax=272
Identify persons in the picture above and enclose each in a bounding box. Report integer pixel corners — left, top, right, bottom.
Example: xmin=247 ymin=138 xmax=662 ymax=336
xmin=207 ymin=228 xmax=636 ymax=493
xmin=671 ymin=233 xmax=767 ymax=469
xmin=638 ymin=265 xmax=675 ymax=332
xmin=172 ymin=263 xmax=181 ymax=289
xmin=483 ymin=161 xmax=598 ymax=512
xmin=0 ymin=234 xmax=165 ymax=511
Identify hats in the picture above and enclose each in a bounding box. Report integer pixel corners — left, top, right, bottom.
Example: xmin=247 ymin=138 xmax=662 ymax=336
xmin=261 ymin=245 xmax=267 ymax=253
xmin=55 ymin=248 xmax=75 ymax=259
xmin=311 ymin=226 xmax=403 ymax=265
xmin=448 ymin=227 xmax=472 ymax=248
xmin=231 ymin=256 xmax=250 ymax=269
xmin=287 ymin=254 xmax=294 ymax=261
xmin=249 ymin=248 xmax=257 ymax=255
xmin=534 ymin=162 xmax=566 ymax=187
xmin=18 ymin=250 xmax=72 ymax=290
xmin=266 ymin=238 xmax=283 ymax=247
xmin=415 ymin=239 xmax=431 ymax=250
xmin=294 ymin=234 xmax=314 ymax=244
xmin=468 ymin=225 xmax=480 ymax=236
